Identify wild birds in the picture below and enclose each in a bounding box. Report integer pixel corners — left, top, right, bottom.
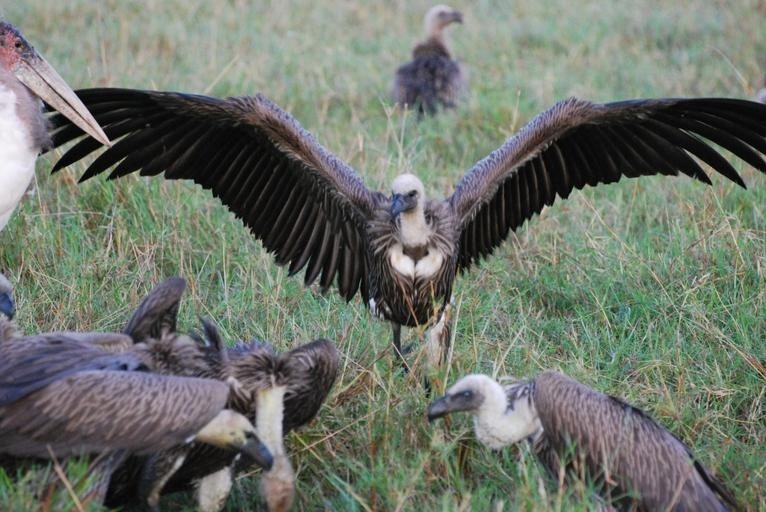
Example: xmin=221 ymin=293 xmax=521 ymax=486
xmin=1 ymin=19 xmax=114 ymax=234
xmin=37 ymin=87 xmax=765 ymax=399
xmin=392 ymin=3 xmax=467 ymax=121
xmin=0 ymin=272 xmax=340 ymax=512
xmin=426 ymin=369 xmax=751 ymax=512
